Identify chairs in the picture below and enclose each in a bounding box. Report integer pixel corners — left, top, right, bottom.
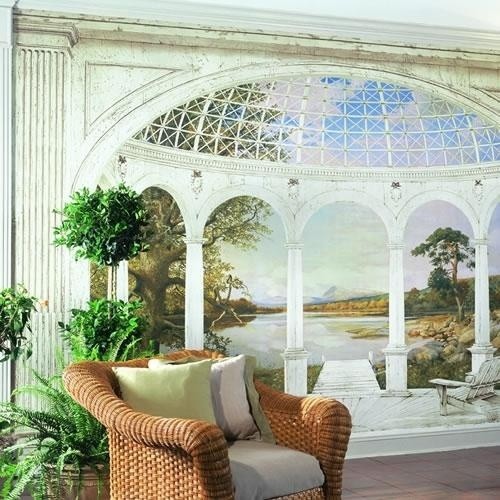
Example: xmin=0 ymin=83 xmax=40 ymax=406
xmin=63 ymin=349 xmax=353 ymax=500
xmin=427 ymin=356 xmax=500 ymax=416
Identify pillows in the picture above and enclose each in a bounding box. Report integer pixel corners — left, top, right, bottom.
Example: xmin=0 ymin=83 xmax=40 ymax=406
xmin=111 ymin=353 xmax=277 ymax=447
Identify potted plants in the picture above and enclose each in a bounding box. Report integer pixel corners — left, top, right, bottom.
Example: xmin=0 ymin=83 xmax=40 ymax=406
xmin=0 ymin=182 xmax=157 ymax=500
